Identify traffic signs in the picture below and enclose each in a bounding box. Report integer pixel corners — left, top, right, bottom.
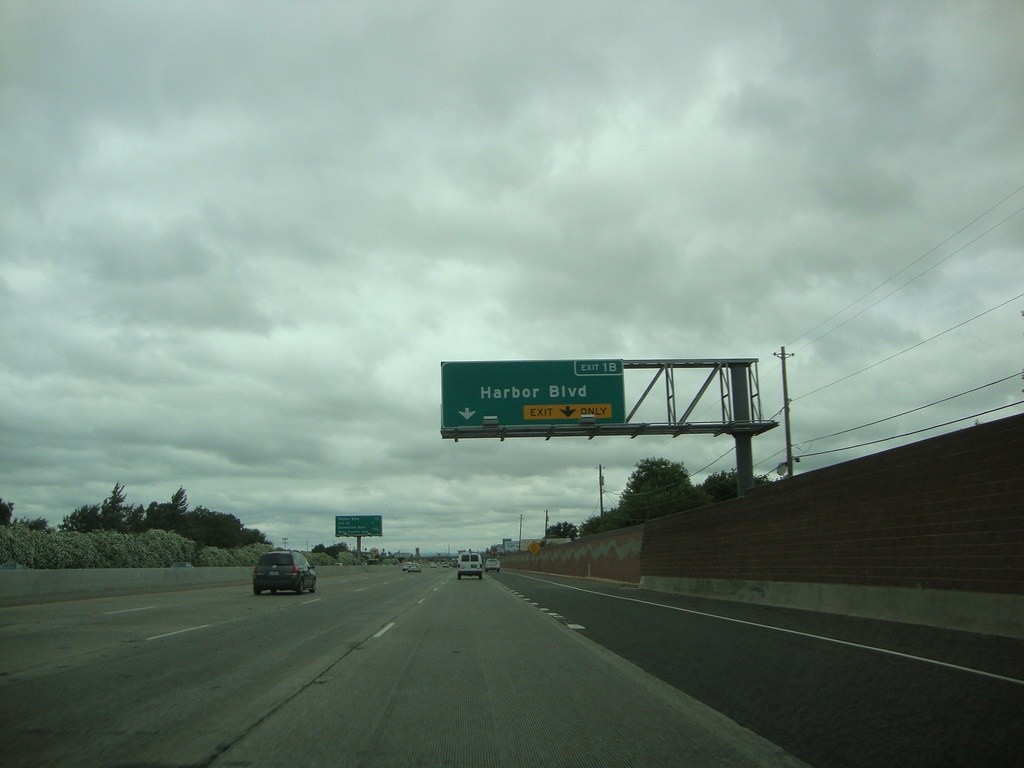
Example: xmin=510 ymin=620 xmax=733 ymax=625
xmin=439 ymin=360 xmax=626 ymax=424
xmin=335 ymin=515 xmax=382 ymax=536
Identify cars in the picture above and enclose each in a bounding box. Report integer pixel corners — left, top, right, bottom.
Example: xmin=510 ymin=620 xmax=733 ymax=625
xmin=485 ymin=558 xmax=500 ymax=572
xmin=402 ymin=562 xmax=412 ymax=571
xmin=382 ymin=563 xmax=402 ymax=568
xmin=426 ymin=560 xmax=457 ymax=569
xmin=253 ymin=550 xmax=316 ymax=595
xmin=457 ymin=548 xmax=483 ymax=580
xmin=407 ymin=563 xmax=421 ymax=573
xmin=334 ymin=562 xmax=343 ymax=566
xmin=360 ymin=562 xmax=367 ymax=567
